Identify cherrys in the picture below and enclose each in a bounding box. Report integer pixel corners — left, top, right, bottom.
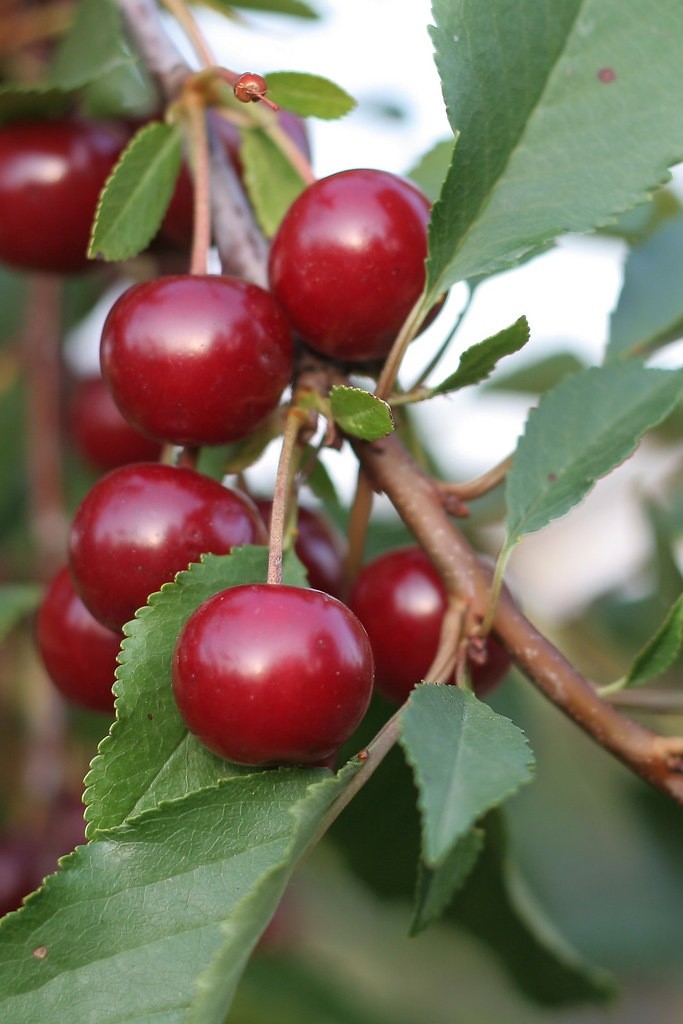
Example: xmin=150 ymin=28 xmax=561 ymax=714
xmin=0 ymin=74 xmax=518 ymax=767
